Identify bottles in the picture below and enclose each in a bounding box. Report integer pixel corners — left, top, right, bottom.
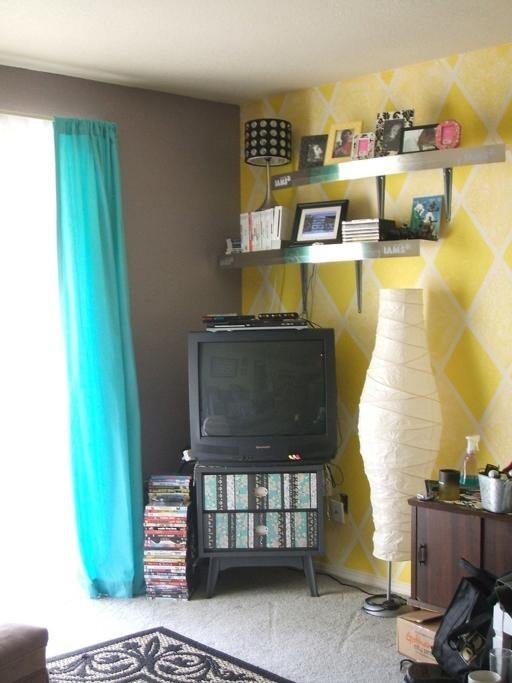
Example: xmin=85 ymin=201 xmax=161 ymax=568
xmin=437 ymin=467 xmax=460 ymax=501
xmin=459 ymin=434 xmax=488 ymax=493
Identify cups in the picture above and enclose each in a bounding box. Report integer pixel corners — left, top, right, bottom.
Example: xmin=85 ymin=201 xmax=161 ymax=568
xmin=467 ymin=669 xmax=501 ymax=683
xmin=486 ymin=647 xmax=511 ymax=683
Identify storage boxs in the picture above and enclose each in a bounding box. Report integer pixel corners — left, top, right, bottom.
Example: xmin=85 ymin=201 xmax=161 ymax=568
xmin=396 ymin=609 xmax=445 ymax=666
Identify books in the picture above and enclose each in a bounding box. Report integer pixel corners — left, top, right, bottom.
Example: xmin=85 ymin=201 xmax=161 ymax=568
xmin=240 ymin=205 xmax=289 ymax=252
xmin=143 ymin=472 xmax=194 ymax=602
xmin=340 ymin=219 xmax=395 ymax=243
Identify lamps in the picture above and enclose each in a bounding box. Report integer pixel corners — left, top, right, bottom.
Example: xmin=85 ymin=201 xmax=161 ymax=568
xmin=243 ymin=117 xmax=294 ymax=212
xmin=354 ymin=286 xmax=446 ymax=616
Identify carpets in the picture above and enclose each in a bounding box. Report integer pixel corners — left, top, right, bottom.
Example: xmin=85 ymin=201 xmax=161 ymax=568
xmin=41 ymin=623 xmax=296 ymax=681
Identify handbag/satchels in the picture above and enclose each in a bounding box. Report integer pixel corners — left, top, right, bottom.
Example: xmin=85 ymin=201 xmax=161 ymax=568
xmin=430 ymin=556 xmax=503 ymax=682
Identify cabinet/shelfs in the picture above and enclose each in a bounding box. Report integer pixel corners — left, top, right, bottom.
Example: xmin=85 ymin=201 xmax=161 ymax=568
xmin=192 ymin=463 xmax=330 ymax=599
xmin=405 ymin=494 xmax=512 ymax=612
xmin=219 ymin=144 xmax=509 ymax=317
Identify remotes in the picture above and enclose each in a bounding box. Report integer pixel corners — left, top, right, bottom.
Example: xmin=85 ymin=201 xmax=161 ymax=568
xmin=205 ymin=312 xmax=238 ymax=317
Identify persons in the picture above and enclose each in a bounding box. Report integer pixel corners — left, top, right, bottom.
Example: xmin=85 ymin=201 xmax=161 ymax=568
xmin=411 ymin=204 xmax=424 ymax=231
xmin=404 ymin=127 xmax=436 ymax=152
xmin=334 ymin=129 xmax=352 ymax=155
xmin=313 ymin=143 xmax=323 ymax=160
xmin=425 ymin=208 xmax=436 ymax=237
xmin=386 ymin=122 xmax=403 ymax=154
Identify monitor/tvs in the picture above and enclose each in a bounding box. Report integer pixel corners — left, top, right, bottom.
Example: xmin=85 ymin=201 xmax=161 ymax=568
xmin=186 ymin=327 xmax=339 ymax=468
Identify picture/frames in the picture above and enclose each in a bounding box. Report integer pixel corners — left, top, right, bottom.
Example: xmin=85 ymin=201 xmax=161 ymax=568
xmin=288 ymin=198 xmax=349 ymax=248
xmin=298 ymin=107 xmax=460 ymax=169
